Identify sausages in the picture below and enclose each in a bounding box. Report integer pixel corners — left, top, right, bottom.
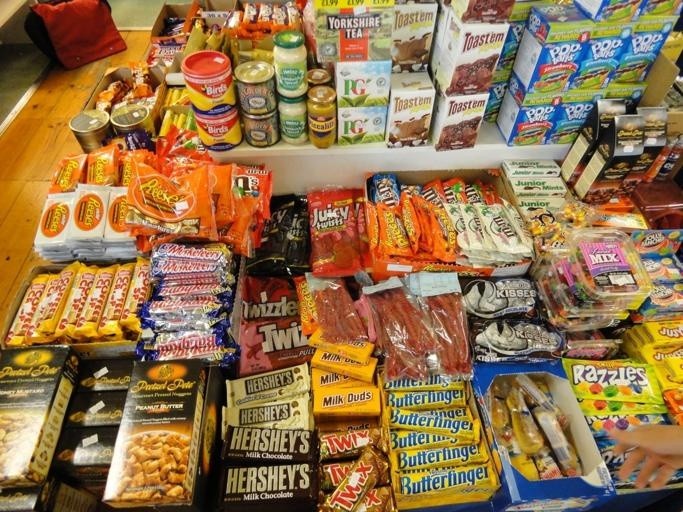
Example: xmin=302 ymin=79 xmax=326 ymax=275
xmin=368 ymin=285 xmax=437 ymax=383
xmin=310 ymin=279 xmax=367 ymax=345
xmin=427 ymin=291 xmax=469 ymax=375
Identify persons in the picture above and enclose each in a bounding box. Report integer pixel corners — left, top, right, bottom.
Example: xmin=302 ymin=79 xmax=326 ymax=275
xmin=607 ymin=421 xmax=683 ymax=492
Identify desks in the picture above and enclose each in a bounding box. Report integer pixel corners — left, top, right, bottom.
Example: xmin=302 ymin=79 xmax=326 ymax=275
xmin=207 ymin=122 xmax=574 ymax=199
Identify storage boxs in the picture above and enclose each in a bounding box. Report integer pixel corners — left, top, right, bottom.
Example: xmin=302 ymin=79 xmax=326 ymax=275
xmin=1 ymin=264 xmax=138 ymax=362
xmin=81 ymin=65 xmax=167 ymax=137
xmin=195 ymin=0 xmax=240 ymax=28
xmin=150 ymin=1 xmax=201 ymax=45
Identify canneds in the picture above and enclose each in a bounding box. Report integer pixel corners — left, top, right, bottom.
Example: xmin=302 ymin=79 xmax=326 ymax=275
xmin=66 ymin=102 xmax=158 ymax=154
xmin=179 ymin=30 xmax=336 ymax=153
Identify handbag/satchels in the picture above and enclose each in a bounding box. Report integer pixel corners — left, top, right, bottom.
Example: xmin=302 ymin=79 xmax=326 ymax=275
xmin=25 ymin=1 xmax=127 ymax=69
